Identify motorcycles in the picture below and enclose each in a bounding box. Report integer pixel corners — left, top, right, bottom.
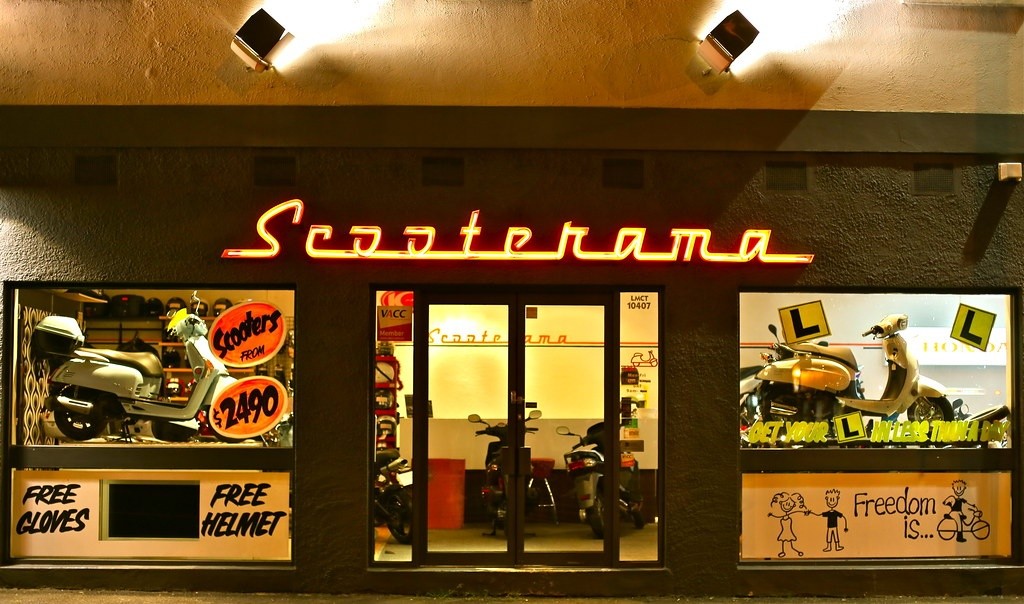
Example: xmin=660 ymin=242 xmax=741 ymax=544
xmin=373 ymin=448 xmax=413 ymax=545
xmin=555 ymin=422 xmax=645 ymax=540
xmin=468 ymin=410 xmax=542 ymax=538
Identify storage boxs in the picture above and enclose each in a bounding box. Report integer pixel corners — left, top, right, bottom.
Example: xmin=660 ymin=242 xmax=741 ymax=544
xmin=110 ymin=294 xmax=144 ymax=316
xmin=84 ymin=294 xmax=111 ymax=318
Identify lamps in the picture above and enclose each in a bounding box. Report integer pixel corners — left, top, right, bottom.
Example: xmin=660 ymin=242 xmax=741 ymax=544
xmin=229 ymin=6 xmax=286 ymax=75
xmin=696 ymin=10 xmax=762 ymax=76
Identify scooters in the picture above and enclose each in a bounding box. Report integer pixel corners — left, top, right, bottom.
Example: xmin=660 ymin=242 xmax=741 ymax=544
xmin=30 ymin=290 xmax=246 ymax=444
xmin=757 ymin=313 xmax=955 ymax=443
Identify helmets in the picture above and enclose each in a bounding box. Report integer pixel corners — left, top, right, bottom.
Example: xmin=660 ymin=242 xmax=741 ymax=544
xmin=166 ymin=296 xmax=186 ymax=313
xmin=163 ymin=350 xmax=180 ymax=368
xmin=165 ymin=378 xmax=185 ymax=397
xmin=145 ymin=298 xmax=163 ymax=318
xmin=213 ymin=299 xmax=232 ymax=317
xmin=191 ymin=296 xmax=210 ymax=316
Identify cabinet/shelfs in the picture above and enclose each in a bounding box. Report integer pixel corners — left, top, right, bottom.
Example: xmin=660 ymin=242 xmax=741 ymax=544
xmin=374 ymin=355 xmax=398 ymax=448
xmin=159 ymin=315 xmax=258 ymax=405
